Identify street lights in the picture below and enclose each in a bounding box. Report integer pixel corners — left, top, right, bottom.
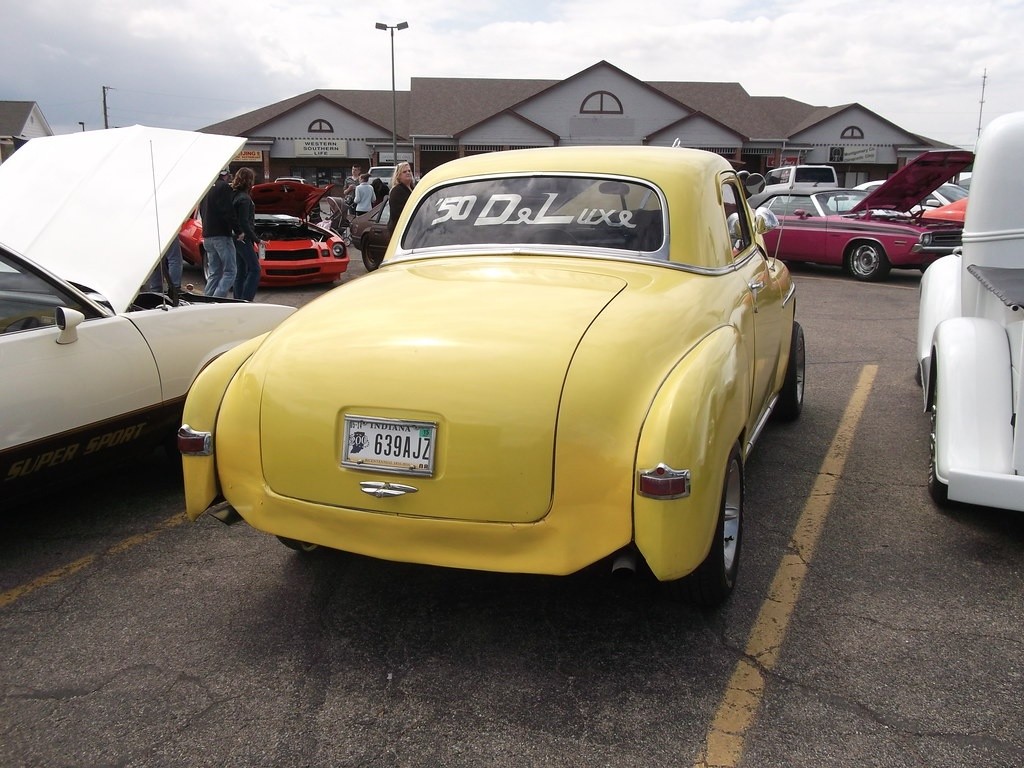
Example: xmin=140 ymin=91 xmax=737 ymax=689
xmin=375 ymin=21 xmax=409 ymax=165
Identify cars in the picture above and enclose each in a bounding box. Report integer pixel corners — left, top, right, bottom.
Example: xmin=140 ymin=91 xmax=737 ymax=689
xmin=275 ymin=178 xmax=307 ymax=184
xmin=756 ymin=149 xmax=975 ymax=281
xmin=368 ymin=166 xmax=396 ymax=190
xmin=914 ymin=111 xmax=1023 ymax=513
xmin=0 ymin=123 xmax=300 ymax=499
xmin=179 ymin=182 xmax=351 ymax=289
xmin=176 ymin=143 xmax=807 ymax=608
xmin=349 ymin=191 xmax=391 ymax=272
xmin=829 ymin=178 xmax=969 ymax=217
xmin=764 ymin=165 xmax=839 ymax=195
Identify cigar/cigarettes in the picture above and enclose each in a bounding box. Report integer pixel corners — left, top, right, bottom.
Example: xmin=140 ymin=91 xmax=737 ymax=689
xmin=241 ymin=240 xmax=246 ymax=244
xmin=268 ymin=239 xmax=270 ymax=243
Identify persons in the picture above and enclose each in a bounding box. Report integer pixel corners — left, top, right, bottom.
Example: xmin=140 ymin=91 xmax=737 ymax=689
xmin=200 ymin=165 xmax=237 ymax=297
xmin=149 ymin=235 xmax=185 ymax=294
xmin=387 ymin=162 xmax=414 ymax=242
xmin=231 ymin=167 xmax=270 ymax=301
xmin=343 ymin=164 xmax=376 ymax=217
